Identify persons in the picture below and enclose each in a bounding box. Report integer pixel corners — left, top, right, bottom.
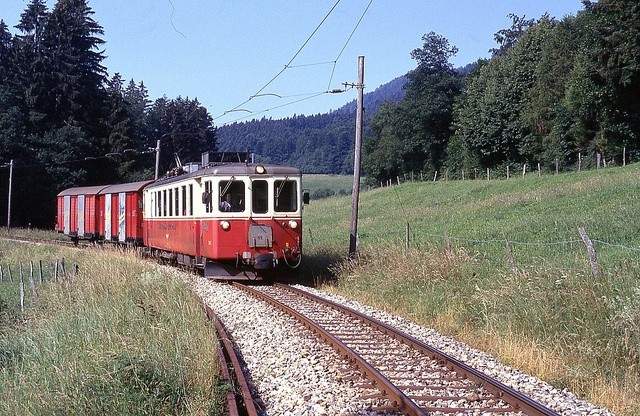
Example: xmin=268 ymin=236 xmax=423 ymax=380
xmin=221 ymin=194 xmax=235 ymax=211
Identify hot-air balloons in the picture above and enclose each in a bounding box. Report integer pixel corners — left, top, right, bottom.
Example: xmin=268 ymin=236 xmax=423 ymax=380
xmin=54 ymin=149 xmax=311 ymax=284
